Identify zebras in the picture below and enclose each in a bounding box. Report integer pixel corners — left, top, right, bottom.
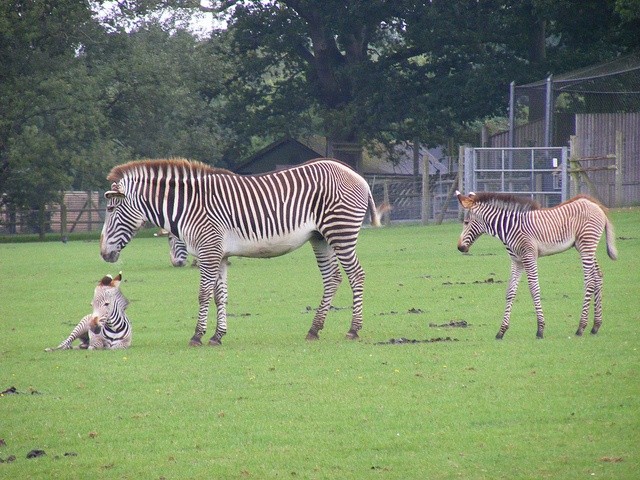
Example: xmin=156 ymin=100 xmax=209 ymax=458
xmin=153 ymin=228 xmax=231 ymax=268
xmin=44 ymin=271 xmax=132 ymax=353
xmin=99 ymin=156 xmax=394 ymax=347
xmin=454 ymin=189 xmax=617 ymax=339
